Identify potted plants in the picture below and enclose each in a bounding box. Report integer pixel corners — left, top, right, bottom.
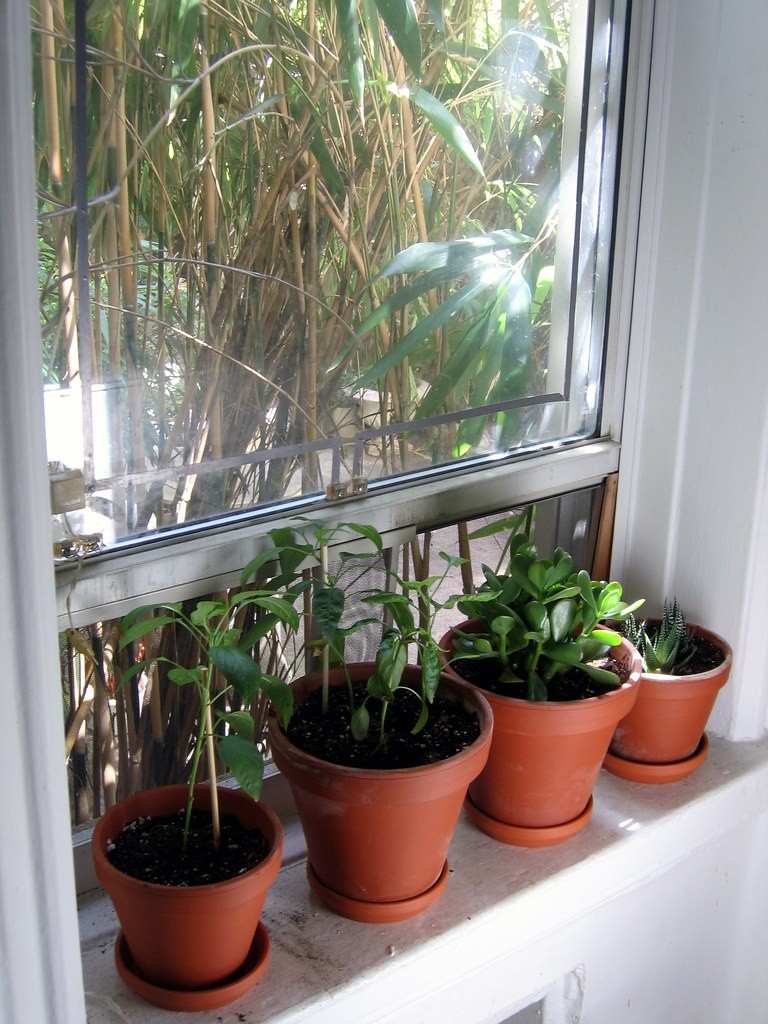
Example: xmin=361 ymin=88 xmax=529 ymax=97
xmin=437 ymin=502 xmax=646 ymax=847
xmin=92 ymin=589 xmax=285 ymax=1011
xmin=242 ymin=515 xmax=494 ymax=923
xmin=601 ymin=597 xmax=734 ymax=784
